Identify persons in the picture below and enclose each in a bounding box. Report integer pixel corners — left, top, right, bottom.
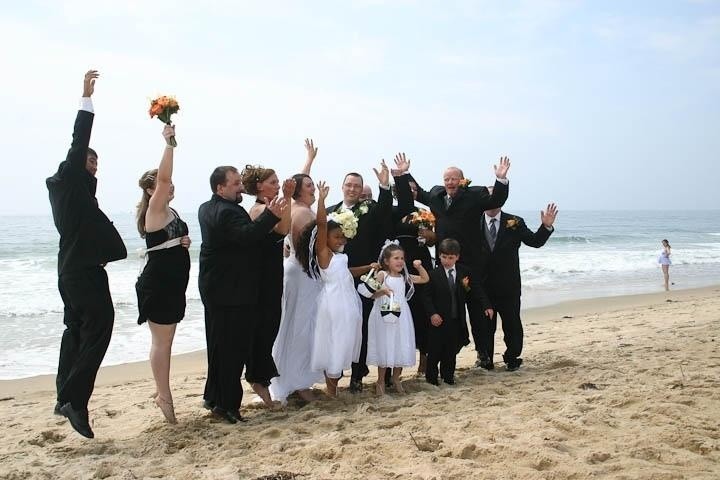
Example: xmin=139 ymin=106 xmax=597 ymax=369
xmin=657 ymin=239 xmax=672 ymax=291
xmin=465 ymin=186 xmax=559 ymax=373
xmin=135 ymin=122 xmax=191 ymax=425
xmin=45 ymin=70 xmax=129 ymax=442
xmin=196 ymin=165 xmax=290 ymax=426
xmin=273 ymin=138 xmax=320 ymax=404
xmin=296 ymin=181 xmax=382 ymax=397
xmin=358 ymin=151 xmax=510 ymax=395
xmin=324 ymin=158 xmax=392 ymax=394
xmin=238 ymin=165 xmax=298 ymax=410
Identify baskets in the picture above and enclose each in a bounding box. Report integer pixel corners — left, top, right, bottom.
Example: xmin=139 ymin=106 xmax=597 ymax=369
xmin=357 ymin=267 xmax=378 ymax=298
xmin=380 ymin=292 xmax=401 ymax=324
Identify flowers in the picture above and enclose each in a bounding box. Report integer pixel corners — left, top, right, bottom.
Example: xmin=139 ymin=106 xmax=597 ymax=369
xmin=461 ymin=276 xmax=471 ymax=290
xmin=381 ymin=302 xmax=402 ymax=314
xmin=505 ymin=218 xmax=521 ymax=231
xmin=359 ymin=272 xmax=384 ymax=292
xmin=335 ymin=208 xmax=358 ymax=242
xmin=353 ymin=199 xmax=371 ymax=216
xmin=148 ymin=96 xmax=185 ymax=149
xmin=457 ymin=177 xmax=472 ymax=193
xmin=401 ymin=208 xmax=437 ymax=245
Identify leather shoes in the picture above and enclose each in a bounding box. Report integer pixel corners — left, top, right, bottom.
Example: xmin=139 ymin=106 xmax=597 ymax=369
xmin=503 ymin=358 xmax=522 ymax=371
xmin=55 ymin=402 xmax=94 ymax=439
xmin=204 ymin=400 xmax=246 ymax=424
xmin=443 ymin=377 xmax=455 ymax=385
xmin=473 ymin=359 xmax=494 ymax=370
xmin=427 ymin=377 xmax=440 ymax=386
